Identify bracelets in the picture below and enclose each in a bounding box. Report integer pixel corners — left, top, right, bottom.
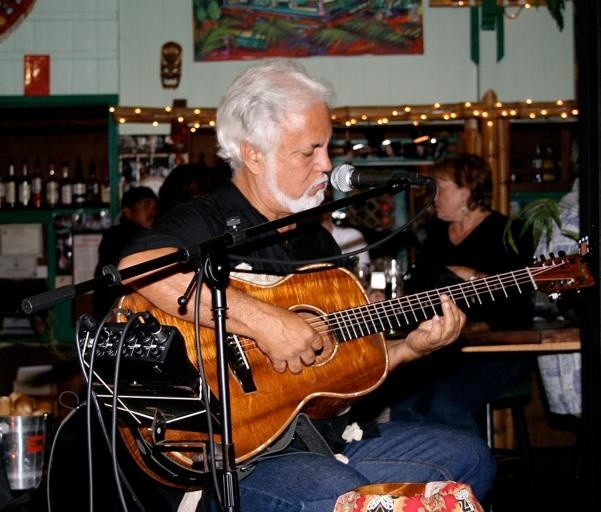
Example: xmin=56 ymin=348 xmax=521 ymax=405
xmin=469 ymin=270 xmax=486 ymax=282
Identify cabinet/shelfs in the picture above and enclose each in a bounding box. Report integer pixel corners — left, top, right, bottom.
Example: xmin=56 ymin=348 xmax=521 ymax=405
xmin=0 ymin=86 xmax=591 ymax=451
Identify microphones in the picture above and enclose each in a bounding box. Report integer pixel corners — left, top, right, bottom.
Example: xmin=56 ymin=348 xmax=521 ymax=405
xmin=330 ymin=164 xmax=430 ymax=193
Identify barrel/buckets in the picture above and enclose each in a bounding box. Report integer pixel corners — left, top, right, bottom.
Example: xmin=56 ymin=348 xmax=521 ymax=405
xmin=0 ymin=412 xmax=49 ymax=491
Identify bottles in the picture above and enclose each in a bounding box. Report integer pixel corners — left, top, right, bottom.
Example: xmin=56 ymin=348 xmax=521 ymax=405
xmin=532 ymin=144 xmax=554 ymax=180
xmin=361 ymin=257 xmax=403 ymax=300
xmin=4 ymin=162 xmax=110 ymax=209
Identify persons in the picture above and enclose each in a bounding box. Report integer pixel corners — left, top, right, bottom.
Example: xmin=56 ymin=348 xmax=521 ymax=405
xmin=155 ymin=126 xmax=234 ymax=230
xmin=530 ymin=157 xmax=584 ymax=428
xmin=389 ymin=149 xmax=534 ymax=441
xmin=92 ymin=185 xmax=159 ymax=323
xmin=113 ymin=54 xmax=495 ymax=511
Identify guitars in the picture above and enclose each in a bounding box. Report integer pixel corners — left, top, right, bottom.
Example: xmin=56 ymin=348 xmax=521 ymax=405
xmin=110 ymin=235 xmax=599 ymax=491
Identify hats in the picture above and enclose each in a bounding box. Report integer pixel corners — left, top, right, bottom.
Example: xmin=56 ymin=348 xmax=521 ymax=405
xmin=122 ymin=185 xmax=158 ymax=207
